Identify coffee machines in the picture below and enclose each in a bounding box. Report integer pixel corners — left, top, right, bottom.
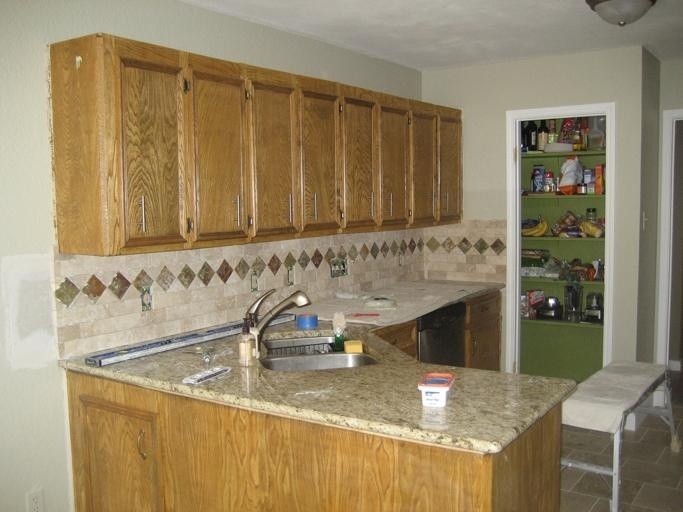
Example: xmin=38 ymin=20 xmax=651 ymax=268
xmin=584 ymin=294 xmax=603 ymax=320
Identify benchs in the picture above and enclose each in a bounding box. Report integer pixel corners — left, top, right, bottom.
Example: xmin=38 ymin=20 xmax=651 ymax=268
xmin=559 ymin=359 xmax=682 ymax=512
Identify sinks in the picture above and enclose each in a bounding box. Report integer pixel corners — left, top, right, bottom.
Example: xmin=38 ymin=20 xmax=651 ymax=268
xmin=263 ymin=336 xmax=350 ymax=354
xmin=260 ymin=355 xmax=382 ymax=372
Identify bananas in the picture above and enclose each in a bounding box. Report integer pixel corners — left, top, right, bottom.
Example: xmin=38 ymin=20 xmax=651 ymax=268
xmin=520 ymin=214 xmax=547 ymax=237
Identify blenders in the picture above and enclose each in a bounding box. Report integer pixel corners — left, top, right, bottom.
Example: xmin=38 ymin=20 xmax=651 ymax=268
xmin=564 ymin=285 xmax=583 ymax=322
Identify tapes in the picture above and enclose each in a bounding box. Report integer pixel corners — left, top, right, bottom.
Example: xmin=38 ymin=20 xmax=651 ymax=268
xmin=297 ymin=314 xmax=318 ymax=329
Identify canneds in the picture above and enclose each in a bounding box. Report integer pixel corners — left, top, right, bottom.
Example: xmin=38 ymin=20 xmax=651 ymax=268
xmin=577 ymin=183 xmax=587 ymax=194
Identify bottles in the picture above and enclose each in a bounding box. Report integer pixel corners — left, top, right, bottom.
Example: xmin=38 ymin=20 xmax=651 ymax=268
xmin=521 ymin=119 xmax=558 ymax=151
xmin=586 ymin=208 xmax=597 ymax=220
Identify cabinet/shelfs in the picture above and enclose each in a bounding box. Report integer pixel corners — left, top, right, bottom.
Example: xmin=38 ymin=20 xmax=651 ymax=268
xmin=51 ymin=33 xmax=191 ymax=257
xmin=519 ymin=149 xmax=606 ymax=330
xmin=461 ymin=289 xmax=502 ymax=372
xmin=67 ymin=370 xmax=165 ymax=512
xmin=370 ymin=318 xmax=418 ymax=360
xmin=187 ymin=51 xmax=247 ymax=250
xmin=246 ymin=63 xmax=463 ymax=243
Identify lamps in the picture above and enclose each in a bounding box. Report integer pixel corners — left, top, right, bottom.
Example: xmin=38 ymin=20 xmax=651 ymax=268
xmin=586 ymin=0 xmax=657 ymax=26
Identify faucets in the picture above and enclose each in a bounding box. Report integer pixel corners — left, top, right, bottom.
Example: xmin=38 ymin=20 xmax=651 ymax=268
xmin=257 ymin=290 xmax=311 ymax=335
